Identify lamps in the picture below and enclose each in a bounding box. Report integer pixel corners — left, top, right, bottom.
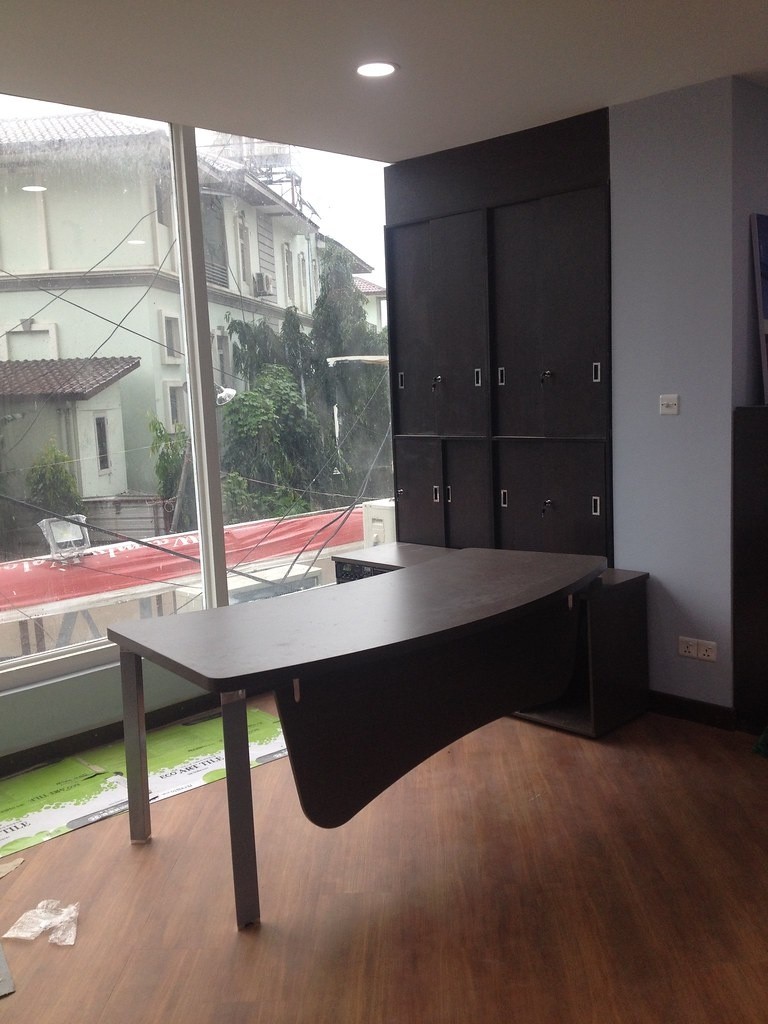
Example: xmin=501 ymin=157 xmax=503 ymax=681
xmin=354 ymin=32 xmax=395 ymax=77
xmin=20 ymin=174 xmax=46 ymax=192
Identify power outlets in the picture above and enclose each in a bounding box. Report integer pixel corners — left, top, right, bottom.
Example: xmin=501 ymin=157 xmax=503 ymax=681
xmin=696 ymin=640 xmax=717 ymax=663
xmin=678 ymin=636 xmax=698 ymax=659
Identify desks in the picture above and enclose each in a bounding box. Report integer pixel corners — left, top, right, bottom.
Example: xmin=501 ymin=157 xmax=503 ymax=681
xmin=105 ymin=551 xmax=608 ymax=928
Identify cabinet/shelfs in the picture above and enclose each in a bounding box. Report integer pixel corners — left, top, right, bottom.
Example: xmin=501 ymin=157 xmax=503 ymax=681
xmin=329 ymin=107 xmax=650 ymax=740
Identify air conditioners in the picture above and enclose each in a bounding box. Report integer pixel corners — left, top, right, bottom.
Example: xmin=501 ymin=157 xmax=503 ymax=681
xmin=254 ymin=272 xmax=273 ymax=295
